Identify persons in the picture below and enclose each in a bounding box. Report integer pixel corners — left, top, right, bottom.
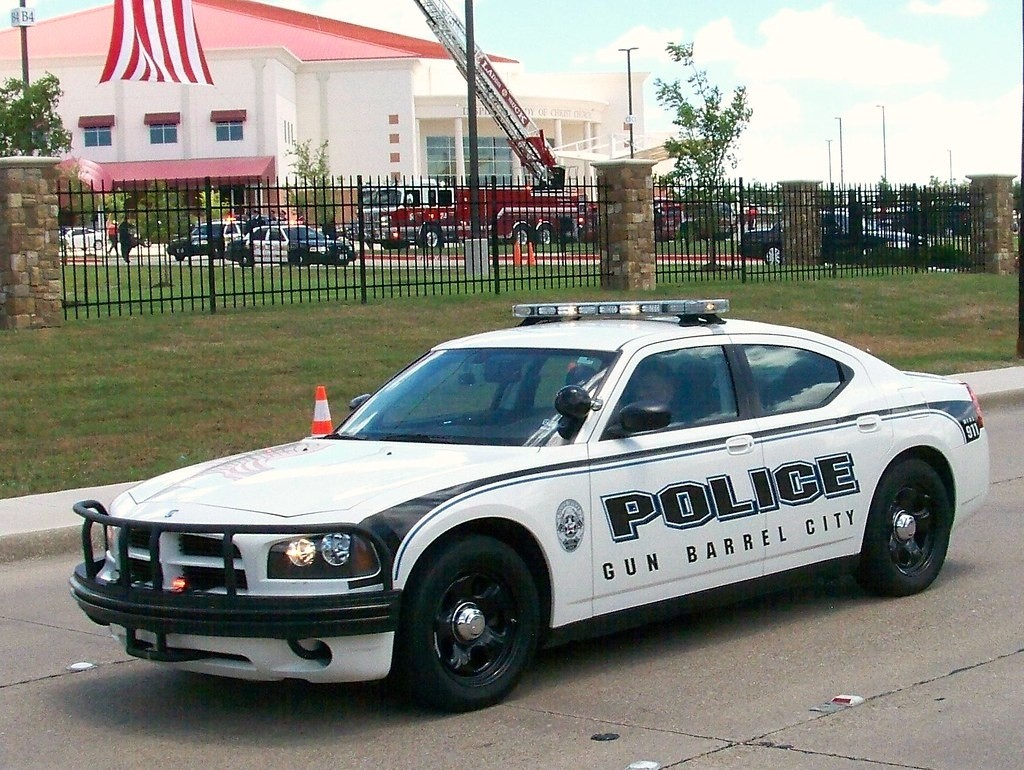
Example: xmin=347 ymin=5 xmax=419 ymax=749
xmin=634 ymin=361 xmax=698 ymax=424
xmin=746 ymin=207 xmax=758 ymax=229
xmin=107 ymin=216 xmax=136 ymax=264
xmin=244 ymin=210 xmax=287 ymax=236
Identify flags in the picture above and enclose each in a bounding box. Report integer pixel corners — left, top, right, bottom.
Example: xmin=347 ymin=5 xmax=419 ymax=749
xmin=96 ymin=0 xmax=215 ymax=87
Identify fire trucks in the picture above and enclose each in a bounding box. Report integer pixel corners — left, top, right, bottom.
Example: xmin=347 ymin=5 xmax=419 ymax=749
xmin=355 ymin=1 xmax=593 ymax=252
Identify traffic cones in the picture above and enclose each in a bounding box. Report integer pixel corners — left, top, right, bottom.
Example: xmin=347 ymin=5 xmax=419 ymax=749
xmin=526 ymin=242 xmax=538 ymax=267
xmin=310 ymin=385 xmax=336 ymax=438
xmin=510 ymin=241 xmax=523 ymax=267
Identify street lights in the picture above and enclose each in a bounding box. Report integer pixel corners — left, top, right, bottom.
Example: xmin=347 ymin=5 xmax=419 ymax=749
xmin=836 ymin=118 xmax=843 ymax=189
xmin=826 ymin=139 xmax=833 ymax=190
xmin=618 ymin=47 xmax=640 ymax=159
xmin=877 ymin=105 xmax=886 ymax=183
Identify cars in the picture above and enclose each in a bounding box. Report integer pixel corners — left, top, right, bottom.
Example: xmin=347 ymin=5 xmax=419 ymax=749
xmin=240 ymin=224 xmax=353 ymax=266
xmin=59 ymin=226 xmax=107 ymax=253
xmin=168 ymin=220 xmax=253 ymax=262
xmin=68 ymin=290 xmax=990 ymax=714
xmin=649 ymin=192 xmax=740 ymax=242
xmin=740 ymin=210 xmax=924 ymax=270
